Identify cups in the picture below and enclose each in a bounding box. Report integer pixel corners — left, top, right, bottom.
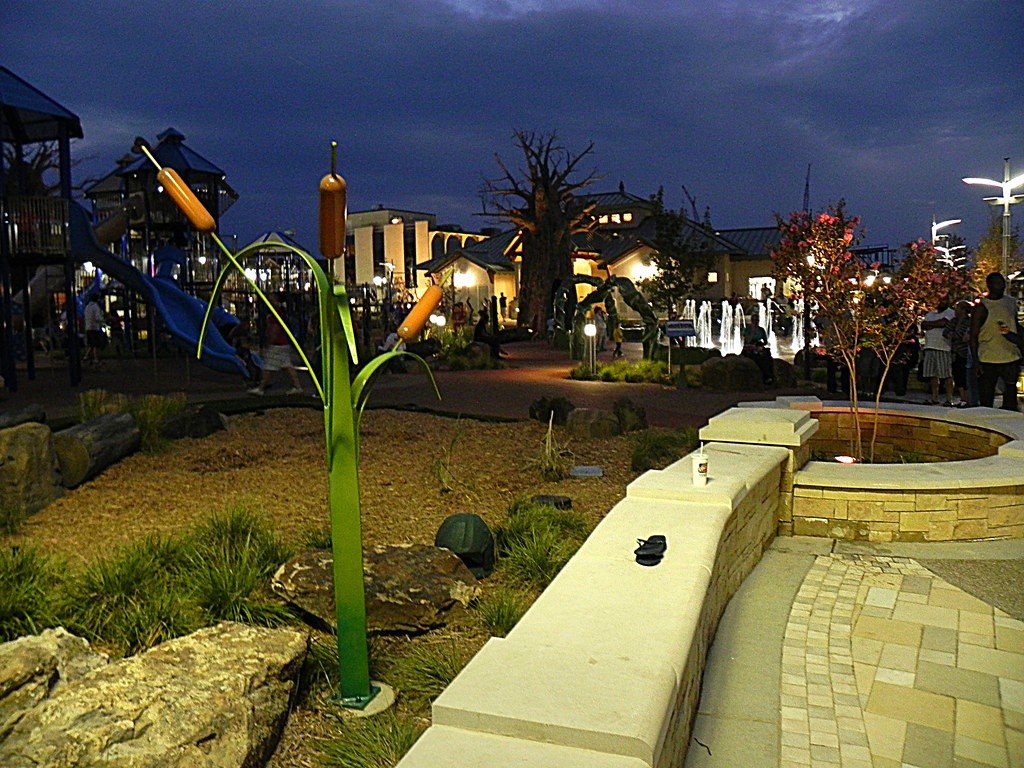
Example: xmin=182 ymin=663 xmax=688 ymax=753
xmin=689 ymin=454 xmax=709 ymax=487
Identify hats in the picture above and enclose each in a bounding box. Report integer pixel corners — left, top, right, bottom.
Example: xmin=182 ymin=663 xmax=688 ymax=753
xmin=267 ymin=295 xmax=278 ymax=307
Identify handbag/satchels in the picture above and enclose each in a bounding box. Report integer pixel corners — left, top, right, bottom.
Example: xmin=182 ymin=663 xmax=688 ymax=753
xmin=99 ymin=320 xmax=109 ymax=351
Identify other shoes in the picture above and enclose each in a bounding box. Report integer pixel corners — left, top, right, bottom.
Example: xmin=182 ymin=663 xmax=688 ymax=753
xmin=246 ymin=388 xmax=264 ymax=397
xmin=496 ymin=354 xmax=505 ymax=360
xmin=958 ymin=401 xmax=968 ymax=408
xmin=600 ymin=347 xmax=607 ymax=351
xmin=618 ymin=353 xmax=624 ymax=357
xmin=285 ymin=387 xmax=304 ymax=395
xmin=949 ymin=398 xmax=962 ymax=406
xmin=312 ymin=394 xmax=321 ymax=399
xmin=612 ymin=352 xmax=616 ymax=359
xmin=501 ymin=350 xmax=508 ymax=356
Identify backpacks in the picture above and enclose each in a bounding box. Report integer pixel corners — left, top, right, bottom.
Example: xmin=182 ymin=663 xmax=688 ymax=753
xmin=275 ymin=309 xmax=301 ymax=344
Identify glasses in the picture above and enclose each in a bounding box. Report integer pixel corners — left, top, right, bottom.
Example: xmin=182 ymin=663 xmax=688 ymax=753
xmin=753 ymin=319 xmax=759 ymax=321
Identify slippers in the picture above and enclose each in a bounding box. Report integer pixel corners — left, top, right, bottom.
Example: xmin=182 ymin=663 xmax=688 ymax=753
xmin=943 ymin=400 xmax=951 ymax=407
xmin=922 ymin=398 xmax=939 ymax=405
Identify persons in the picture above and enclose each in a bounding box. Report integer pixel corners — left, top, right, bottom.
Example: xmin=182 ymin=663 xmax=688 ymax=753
xmin=248 ymin=295 xmax=322 ymax=398
xmin=842 ymin=272 xmax=1024 ymax=411
xmin=742 ymin=314 xmax=773 ymax=384
xmin=613 ymin=323 xmax=624 ymax=357
xmin=547 ymin=314 xmax=554 ymax=345
xmin=594 ymin=307 xmax=609 ymax=351
xmin=40 ymin=295 xmax=173 ymax=363
xmin=474 ymin=311 xmax=508 ymax=359
xmin=499 ymin=292 xmax=507 ymax=321
xmin=530 ymin=314 xmax=540 ymax=344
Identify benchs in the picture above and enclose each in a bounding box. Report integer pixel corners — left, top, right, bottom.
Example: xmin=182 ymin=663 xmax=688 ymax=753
xmin=878 ymin=368 xmax=929 ymax=390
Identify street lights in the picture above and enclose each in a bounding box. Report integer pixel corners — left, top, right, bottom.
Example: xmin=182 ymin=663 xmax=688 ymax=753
xmin=934 ymin=245 xmax=966 ymax=265
xmin=931 ymin=219 xmax=961 ymax=245
xmin=961 ymin=173 xmax=1024 ymax=297
